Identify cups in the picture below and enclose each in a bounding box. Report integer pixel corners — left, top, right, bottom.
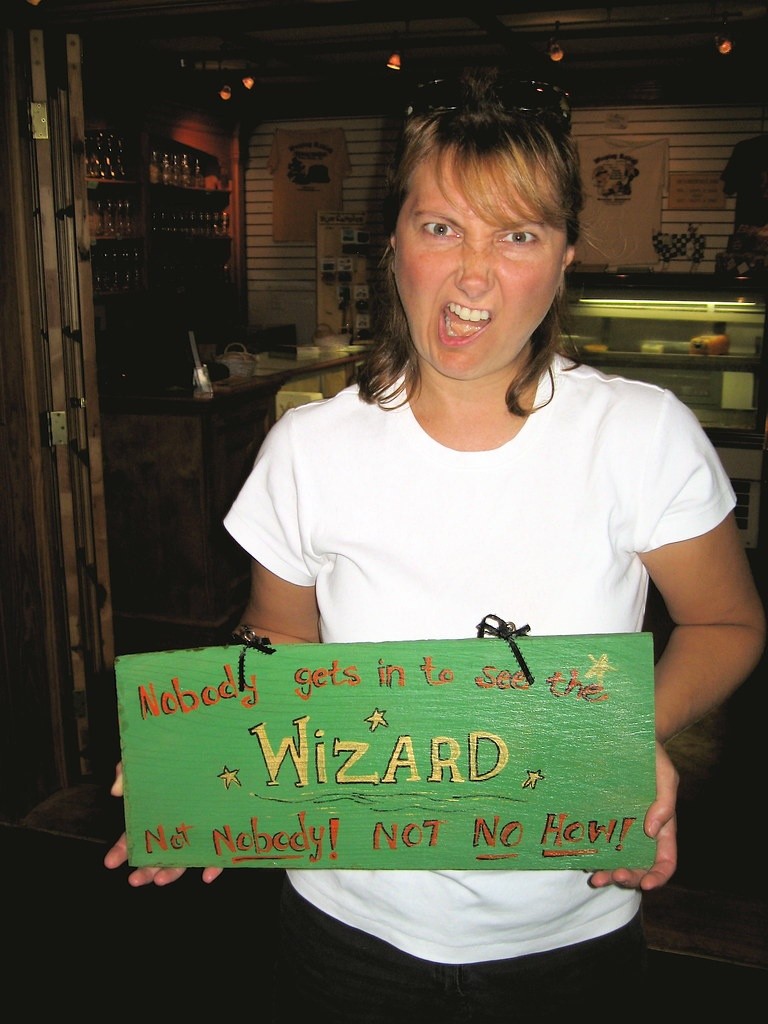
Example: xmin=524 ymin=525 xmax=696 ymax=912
xmin=193 ymin=364 xmax=215 ymax=399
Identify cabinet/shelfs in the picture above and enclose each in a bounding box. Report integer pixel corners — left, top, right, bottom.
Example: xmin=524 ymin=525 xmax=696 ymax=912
xmin=78 ymin=85 xmax=247 ymax=392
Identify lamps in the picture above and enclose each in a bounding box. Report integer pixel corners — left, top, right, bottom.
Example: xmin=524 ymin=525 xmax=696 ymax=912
xmin=387 ymin=54 xmax=401 ymax=71
xmin=550 ymin=21 xmax=564 ymax=62
xmin=220 ymin=74 xmax=254 ymax=100
xmin=713 ymin=18 xmax=732 ymax=53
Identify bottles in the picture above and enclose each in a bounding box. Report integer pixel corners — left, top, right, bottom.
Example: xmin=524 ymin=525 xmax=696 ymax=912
xmin=149 ymin=151 xmax=204 ymax=188
xmin=149 ymin=207 xmax=230 ymax=238
xmin=92 ymin=248 xmax=143 ymax=292
xmin=341 ymin=304 xmax=354 ymax=344
xmin=94 ymin=198 xmax=133 ymax=236
xmin=82 ymin=132 xmax=128 ymax=180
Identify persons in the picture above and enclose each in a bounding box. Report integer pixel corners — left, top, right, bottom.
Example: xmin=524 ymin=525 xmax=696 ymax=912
xmin=104 ymin=65 xmax=768 ymax=1024
